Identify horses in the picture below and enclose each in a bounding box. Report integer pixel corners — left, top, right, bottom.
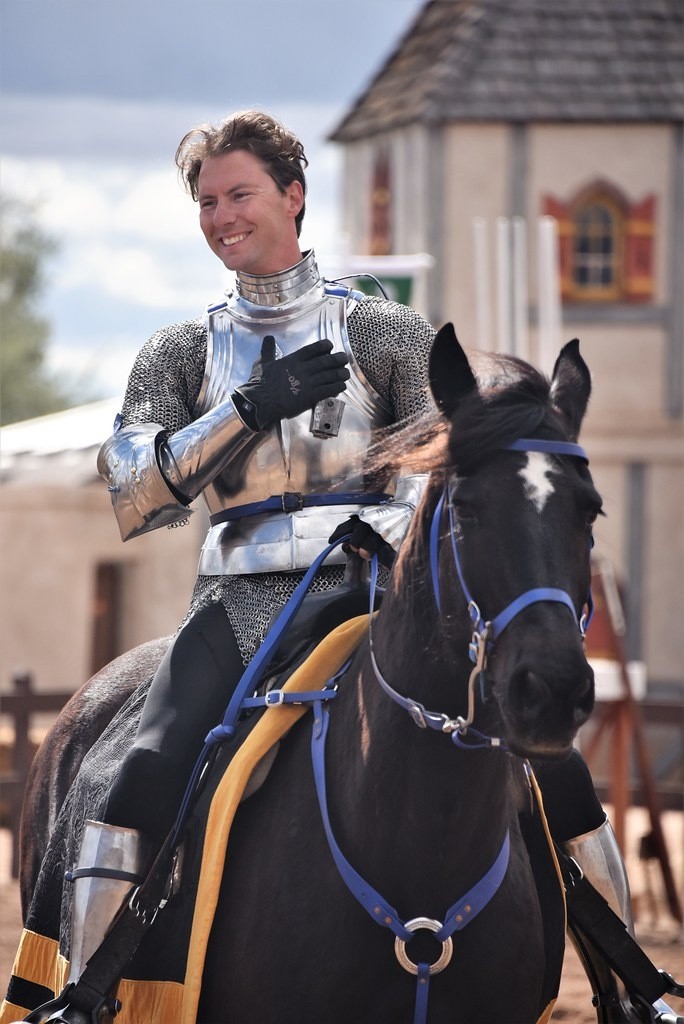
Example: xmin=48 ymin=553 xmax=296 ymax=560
xmin=20 ymin=322 xmax=607 ymax=1024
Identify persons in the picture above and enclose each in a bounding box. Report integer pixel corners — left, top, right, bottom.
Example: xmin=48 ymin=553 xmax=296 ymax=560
xmin=40 ymin=110 xmax=681 ymax=1023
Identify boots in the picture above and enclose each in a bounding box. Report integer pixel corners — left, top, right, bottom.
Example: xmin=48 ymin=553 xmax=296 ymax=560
xmin=45 ymin=819 xmax=156 ymax=1024
xmin=558 ymin=813 xmax=684 ymax=1024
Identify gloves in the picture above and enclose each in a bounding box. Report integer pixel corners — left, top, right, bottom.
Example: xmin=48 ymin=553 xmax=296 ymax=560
xmin=234 ymin=336 xmax=350 ymax=429
